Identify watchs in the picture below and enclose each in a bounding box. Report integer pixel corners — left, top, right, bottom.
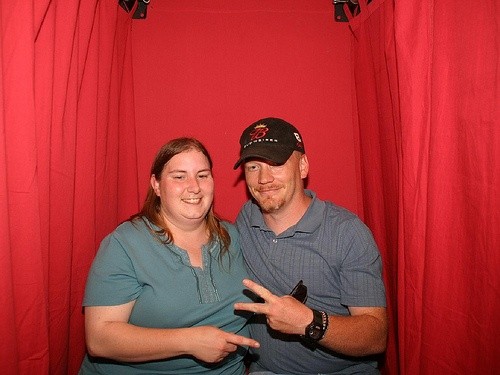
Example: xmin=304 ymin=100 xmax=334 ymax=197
xmin=233 ymin=116 xmax=388 ymax=375
xmin=299 ymin=309 xmax=323 ymax=344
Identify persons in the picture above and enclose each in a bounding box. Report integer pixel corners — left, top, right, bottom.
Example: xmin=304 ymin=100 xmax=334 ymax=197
xmin=79 ymin=137 xmax=260 ymax=375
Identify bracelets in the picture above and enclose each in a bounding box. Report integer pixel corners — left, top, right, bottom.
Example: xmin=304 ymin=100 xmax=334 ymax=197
xmin=319 ymin=310 xmax=330 ymax=340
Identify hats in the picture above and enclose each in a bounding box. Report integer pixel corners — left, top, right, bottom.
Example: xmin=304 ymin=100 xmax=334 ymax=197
xmin=233 ymin=117 xmax=305 ymax=170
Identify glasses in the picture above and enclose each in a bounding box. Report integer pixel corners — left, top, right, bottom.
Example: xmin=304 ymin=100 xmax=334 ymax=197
xmin=289 ymin=279 xmax=307 ymax=304
